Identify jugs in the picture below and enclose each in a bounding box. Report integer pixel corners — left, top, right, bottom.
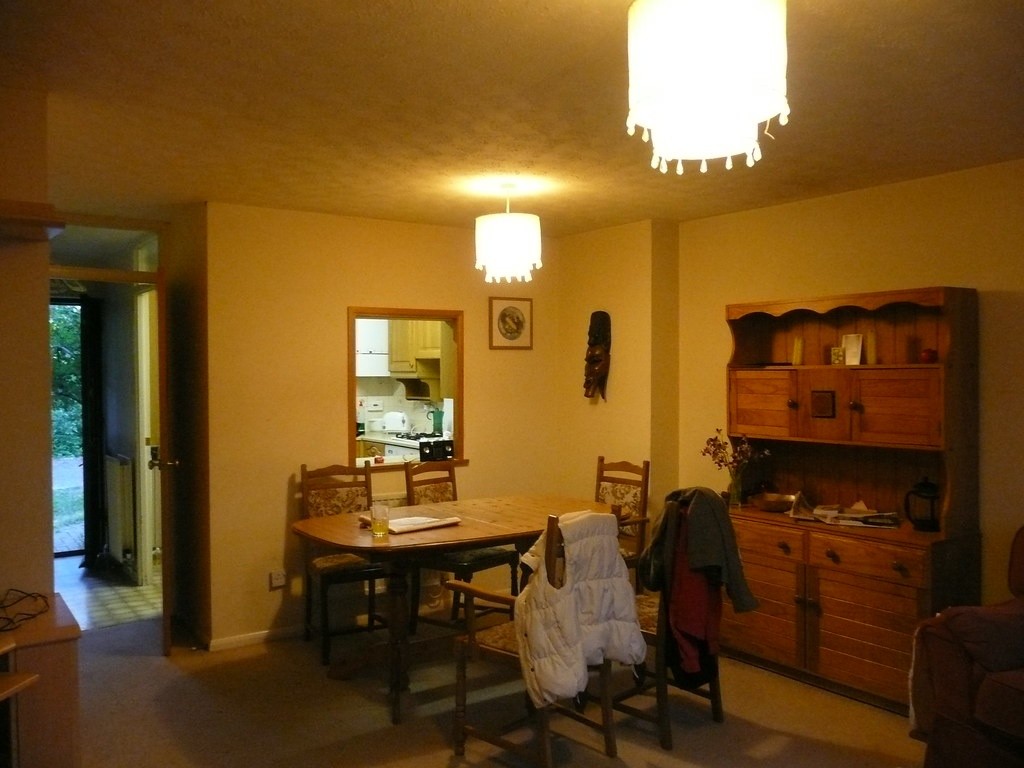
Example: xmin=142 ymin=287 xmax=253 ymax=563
xmin=427 ymin=407 xmax=444 ymax=436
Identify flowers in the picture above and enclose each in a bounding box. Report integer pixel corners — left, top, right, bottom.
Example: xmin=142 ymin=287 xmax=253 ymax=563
xmin=702 ymin=427 xmax=768 ymax=472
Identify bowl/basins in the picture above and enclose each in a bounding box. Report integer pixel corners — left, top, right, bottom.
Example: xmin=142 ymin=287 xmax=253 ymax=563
xmin=749 ymin=494 xmax=796 ymax=513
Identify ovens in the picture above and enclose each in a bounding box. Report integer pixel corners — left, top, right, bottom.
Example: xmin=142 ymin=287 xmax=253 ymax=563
xmin=385 ymin=444 xmax=420 ymax=458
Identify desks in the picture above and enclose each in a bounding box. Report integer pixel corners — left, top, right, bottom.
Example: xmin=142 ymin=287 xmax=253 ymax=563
xmin=296 ymin=497 xmax=649 ymax=724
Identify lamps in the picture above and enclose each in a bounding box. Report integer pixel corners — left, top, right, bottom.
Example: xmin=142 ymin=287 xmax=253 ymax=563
xmin=475 ymin=190 xmax=543 ymax=283
xmin=625 ymin=0 xmax=792 ymax=175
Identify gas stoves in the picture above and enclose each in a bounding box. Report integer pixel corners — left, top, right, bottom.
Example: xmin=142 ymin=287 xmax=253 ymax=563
xmin=392 ymin=430 xmax=432 ymax=443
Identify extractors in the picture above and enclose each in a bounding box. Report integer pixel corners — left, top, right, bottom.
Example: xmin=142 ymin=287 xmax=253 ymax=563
xmin=394 ymin=378 xmax=442 ymax=402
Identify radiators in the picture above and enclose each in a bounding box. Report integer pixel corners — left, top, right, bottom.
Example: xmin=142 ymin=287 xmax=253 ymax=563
xmin=105 ymin=455 xmax=134 ymax=563
xmin=339 ymin=491 xmax=442 ymax=596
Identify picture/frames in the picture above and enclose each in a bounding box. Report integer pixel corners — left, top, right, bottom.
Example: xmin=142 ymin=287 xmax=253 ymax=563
xmin=487 ymin=296 xmax=534 ymax=351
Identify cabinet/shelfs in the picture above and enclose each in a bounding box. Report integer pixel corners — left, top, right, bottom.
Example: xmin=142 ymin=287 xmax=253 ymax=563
xmin=0 ymin=593 xmax=81 ymax=768
xmin=714 ymin=287 xmax=981 ymax=717
xmin=355 ymin=441 xmax=385 ymax=457
xmin=416 ymin=319 xmax=456 ymax=399
xmin=388 ymin=317 xmax=439 ymax=379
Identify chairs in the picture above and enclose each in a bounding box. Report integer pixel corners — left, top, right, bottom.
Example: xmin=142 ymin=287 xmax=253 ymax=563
xmin=403 ymin=460 xmax=520 ymax=633
xmin=594 ymin=455 xmax=650 ymax=594
xmin=574 ymin=487 xmax=732 ymax=751
xmin=301 ymin=461 xmax=389 ymax=644
xmin=456 ymin=507 xmax=622 ymax=768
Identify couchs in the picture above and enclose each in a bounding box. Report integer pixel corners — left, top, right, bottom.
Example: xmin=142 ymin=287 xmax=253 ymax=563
xmin=907 ymin=529 xmax=1024 ymax=768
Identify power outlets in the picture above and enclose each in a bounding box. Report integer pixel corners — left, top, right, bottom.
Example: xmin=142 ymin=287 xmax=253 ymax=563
xmin=270 ymin=570 xmax=289 ymax=587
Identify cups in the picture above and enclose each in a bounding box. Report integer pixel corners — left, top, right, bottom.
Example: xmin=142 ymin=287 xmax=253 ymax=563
xmin=370 ymin=505 xmax=389 ymax=537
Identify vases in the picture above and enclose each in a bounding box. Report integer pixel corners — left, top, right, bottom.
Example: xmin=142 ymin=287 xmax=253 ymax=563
xmin=727 ymin=465 xmax=750 ymax=506
